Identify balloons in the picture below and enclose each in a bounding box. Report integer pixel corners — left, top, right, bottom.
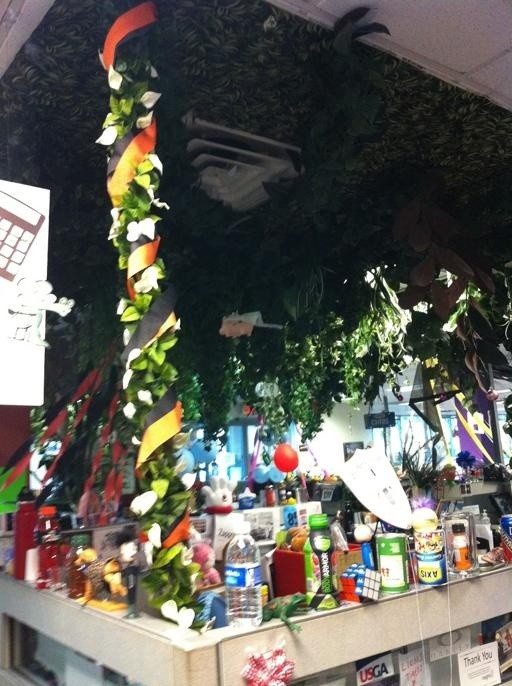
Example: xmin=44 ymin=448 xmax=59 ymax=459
xmin=274 ymin=442 xmax=299 ymax=472
xmin=191 ymin=439 xmax=217 ymax=464
xmin=178 ymin=449 xmax=195 ymax=476
xmin=252 ymin=460 xmax=268 ymax=484
xmin=266 ymin=462 xmax=287 ymax=483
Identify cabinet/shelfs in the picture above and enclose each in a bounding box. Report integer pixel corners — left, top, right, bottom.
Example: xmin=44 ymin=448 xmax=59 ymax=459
xmin=3 ymin=561 xmax=512 ymax=686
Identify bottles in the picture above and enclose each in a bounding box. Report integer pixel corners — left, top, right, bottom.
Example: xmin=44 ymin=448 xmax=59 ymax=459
xmin=68 ymin=535 xmax=89 ymax=596
xmin=460 ymin=480 xmax=466 ymax=494
xmin=499 ymin=514 xmax=512 ymax=563
xmin=361 ymin=543 xmax=375 ymax=571
xmin=13 ymin=485 xmax=37 ymax=580
xmin=374 ymin=533 xmax=410 ymax=594
xmin=304 ymin=514 xmax=340 ymax=610
xmin=412 ymin=530 xmax=448 ymax=587
xmin=33 ymin=505 xmax=61 ymax=586
xmin=223 ymin=521 xmax=263 ymax=629
xmin=278 ymin=481 xmax=287 ymax=505
xmin=451 ymin=523 xmax=470 ymax=570
xmin=264 ymin=485 xmax=276 ymax=506
xmin=466 ymin=480 xmax=472 ymax=493
